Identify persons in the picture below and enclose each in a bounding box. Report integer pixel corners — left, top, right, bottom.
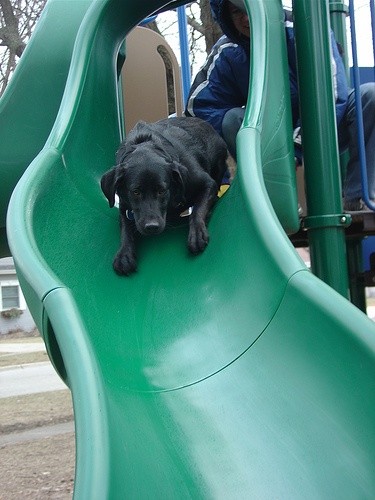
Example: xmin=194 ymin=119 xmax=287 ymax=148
xmin=184 ymin=1 xmax=375 ymax=291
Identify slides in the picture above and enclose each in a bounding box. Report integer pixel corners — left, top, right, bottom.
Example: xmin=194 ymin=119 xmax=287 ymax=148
xmin=2 ymin=0 xmax=375 ymax=500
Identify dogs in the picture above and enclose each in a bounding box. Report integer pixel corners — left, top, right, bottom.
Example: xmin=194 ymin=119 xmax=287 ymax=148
xmin=100 ymin=116 xmax=229 ymax=277
